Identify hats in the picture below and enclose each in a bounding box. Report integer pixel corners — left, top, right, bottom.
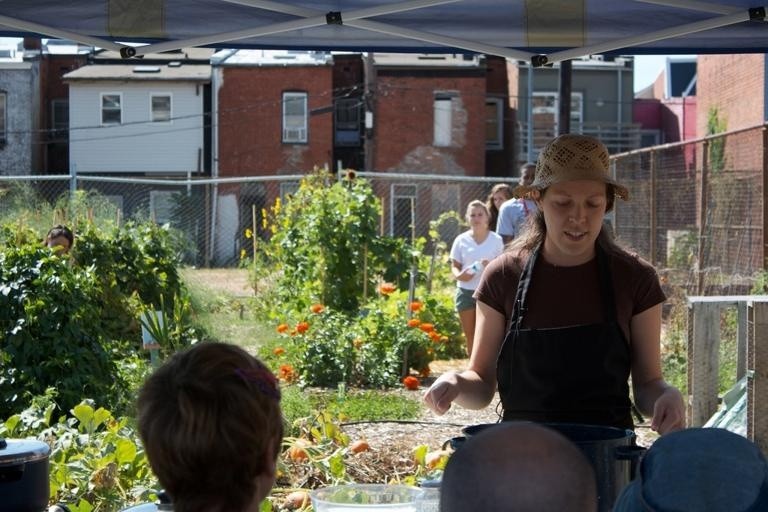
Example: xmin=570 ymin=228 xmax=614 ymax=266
xmin=513 ymin=134 xmax=630 ymax=202
xmin=612 ymin=428 xmax=768 ymax=511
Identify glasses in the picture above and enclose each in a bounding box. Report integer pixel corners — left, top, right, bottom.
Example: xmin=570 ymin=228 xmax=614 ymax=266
xmin=522 ymin=175 xmax=535 ymax=180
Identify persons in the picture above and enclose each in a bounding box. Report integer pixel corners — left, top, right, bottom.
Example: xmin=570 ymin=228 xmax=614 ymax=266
xmin=420 ymin=132 xmax=687 ymax=510
xmin=449 ymin=198 xmax=506 ymax=360
xmin=131 ymin=341 xmax=287 ymax=511
xmin=486 ymin=183 xmax=515 ymax=232
xmin=438 ymin=420 xmax=600 ymax=512
xmin=496 ymin=162 xmax=545 ymax=251
xmin=41 ymin=224 xmax=74 ymax=260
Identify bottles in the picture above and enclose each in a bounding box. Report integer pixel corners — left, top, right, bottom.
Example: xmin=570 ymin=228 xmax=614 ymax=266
xmin=416 ymin=479 xmax=443 ymax=512
xmin=467 ymin=259 xmax=483 ymax=274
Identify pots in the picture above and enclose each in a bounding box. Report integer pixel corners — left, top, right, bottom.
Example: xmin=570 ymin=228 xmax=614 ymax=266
xmin=447 ymin=419 xmax=649 ymax=512
xmin=0 ymin=438 xmax=53 ymax=512
